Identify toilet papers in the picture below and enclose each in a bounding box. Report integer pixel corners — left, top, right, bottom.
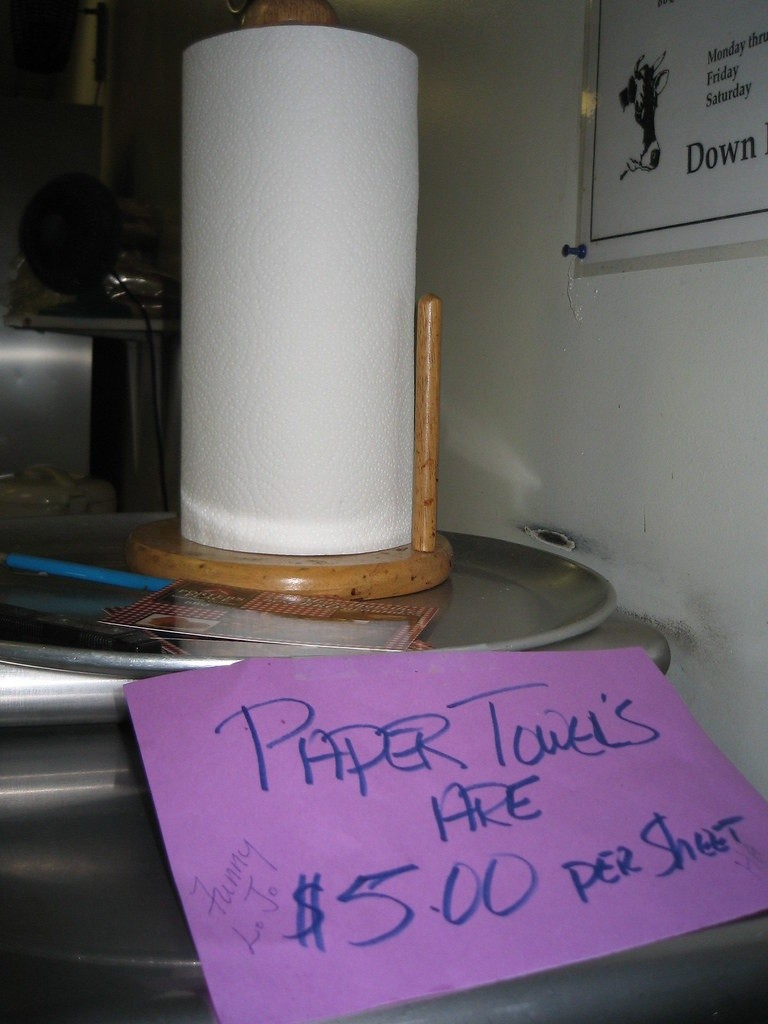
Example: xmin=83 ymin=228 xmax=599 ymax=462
xmin=181 ymin=26 xmax=418 ymax=556
